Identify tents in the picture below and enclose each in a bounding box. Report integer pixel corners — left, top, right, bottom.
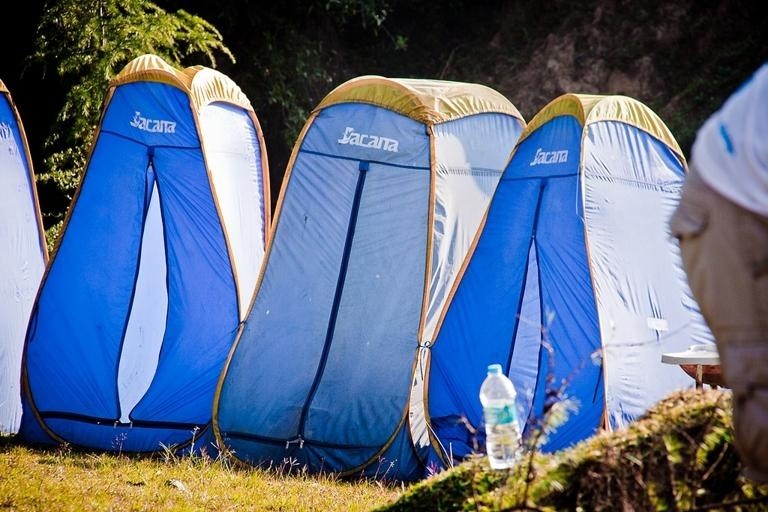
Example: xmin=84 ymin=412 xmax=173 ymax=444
xmin=212 ymin=73 xmax=535 ymax=485
xmin=418 ymin=93 xmax=727 ymax=475
xmin=14 ymin=52 xmax=276 ymax=463
xmin=0 ymin=73 xmax=55 ymax=442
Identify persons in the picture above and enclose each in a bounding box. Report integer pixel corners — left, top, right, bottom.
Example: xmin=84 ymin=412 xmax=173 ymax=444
xmin=668 ymin=62 xmax=768 ymax=487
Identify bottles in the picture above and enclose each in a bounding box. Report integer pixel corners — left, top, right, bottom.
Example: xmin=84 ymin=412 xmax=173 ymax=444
xmin=479 ymin=364 xmax=524 ymax=471
